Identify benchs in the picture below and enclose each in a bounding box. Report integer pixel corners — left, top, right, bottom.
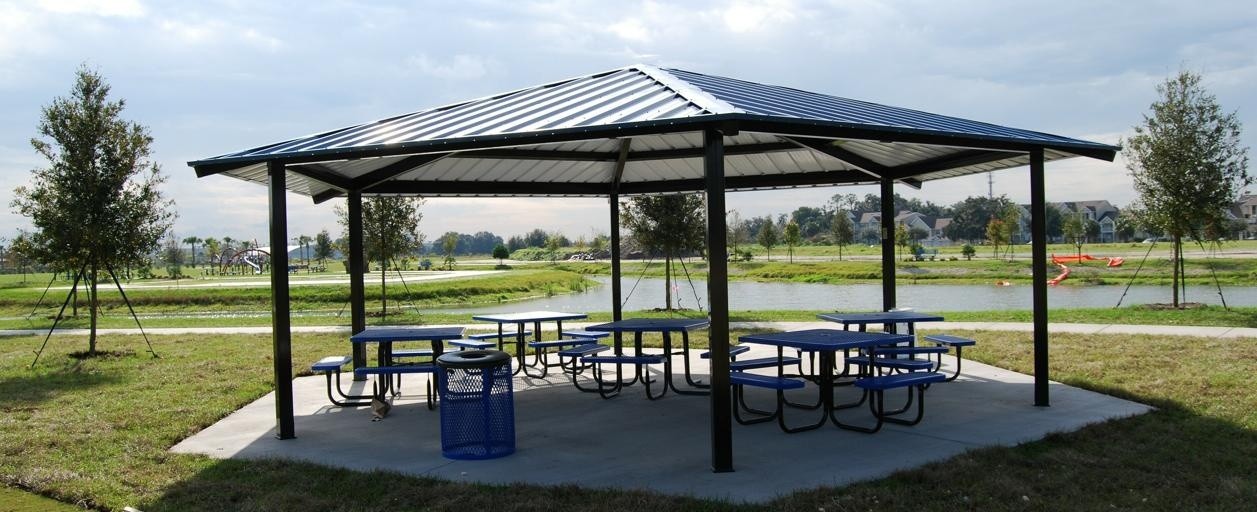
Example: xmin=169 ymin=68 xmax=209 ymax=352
xmin=313 ymin=356 xmax=357 ymax=400
xmin=449 ymin=339 xmax=496 ymax=376
xmin=727 ymin=371 xmax=804 ymax=432
xmin=385 ymin=349 xmax=454 ymax=387
xmin=857 ymin=371 xmax=944 ymax=428
xmin=923 ymin=335 xmax=976 ymax=379
xmin=563 ymin=329 xmax=612 ymax=367
xmin=844 ymin=357 xmax=937 ymax=407
xmin=469 ymin=330 xmax=536 ymax=369
xmin=864 ymin=345 xmax=949 ymax=386
xmin=357 ymin=365 xmax=442 ymax=408
xmin=727 ymin=356 xmax=800 ymax=409
xmin=560 ymin=343 xmax=609 ymax=391
xmin=582 ymin=354 xmax=666 ymax=397
xmin=528 ymin=338 xmax=600 ymax=376
xmin=700 ymin=346 xmax=749 ymax=393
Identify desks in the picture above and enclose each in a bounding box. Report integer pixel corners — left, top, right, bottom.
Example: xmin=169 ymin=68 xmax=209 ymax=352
xmin=471 ymin=313 xmax=712 ymax=400
xmin=349 ymin=328 xmax=467 ymax=401
xmin=737 ymin=328 xmax=918 ymax=421
xmin=817 ymin=311 xmax=947 ymax=391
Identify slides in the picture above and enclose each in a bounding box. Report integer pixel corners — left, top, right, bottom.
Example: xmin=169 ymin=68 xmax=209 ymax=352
xmin=243 ymin=256 xmax=261 ymax=276
